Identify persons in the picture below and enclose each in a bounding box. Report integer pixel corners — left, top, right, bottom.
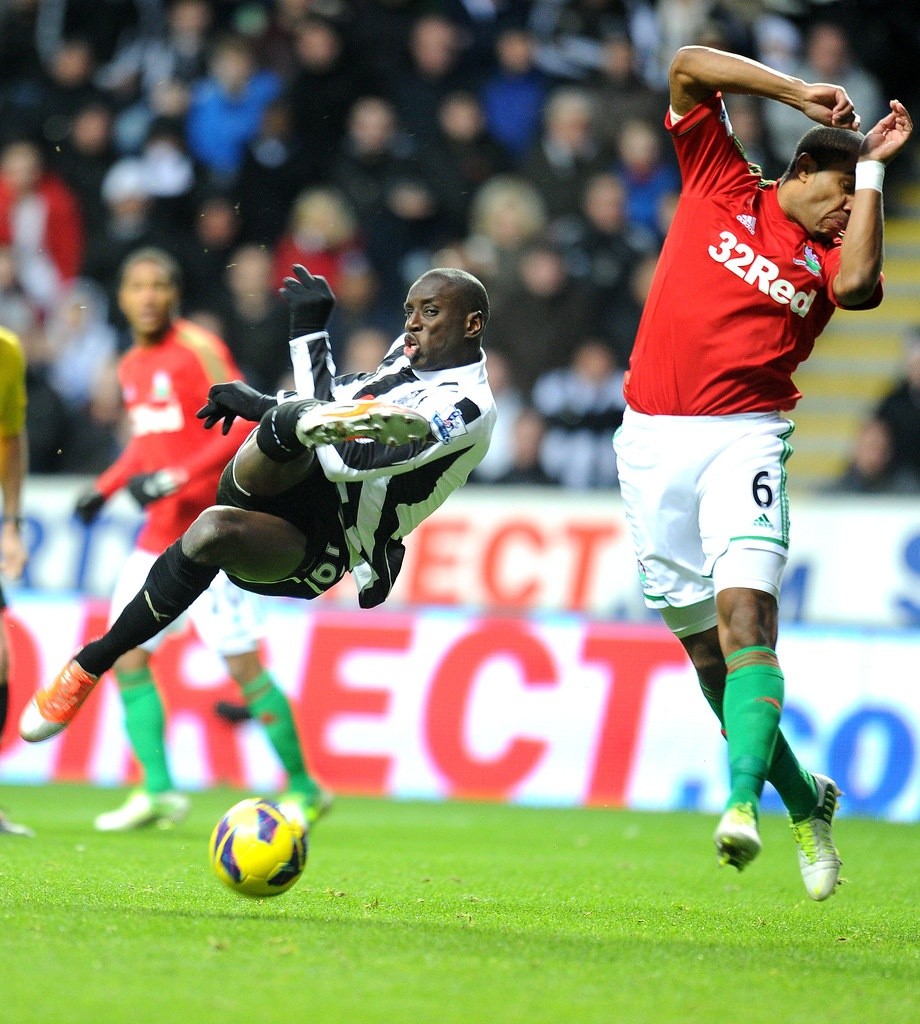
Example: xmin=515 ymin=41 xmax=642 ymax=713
xmin=614 ymin=46 xmax=913 ymax=901
xmin=19 ymin=267 xmax=499 ymax=743
xmin=0 ymin=0 xmax=875 ymax=489
xmin=0 ymin=328 xmax=29 ymax=832
xmin=836 ymin=353 xmax=920 ymax=493
xmin=75 ymin=253 xmax=331 ymax=831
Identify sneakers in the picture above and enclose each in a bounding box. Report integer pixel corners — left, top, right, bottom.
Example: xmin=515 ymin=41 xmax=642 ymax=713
xmin=18 ymin=657 xmax=100 ymax=743
xmin=712 ymin=801 xmax=762 ymax=873
xmin=296 ymin=399 xmax=431 ymax=450
xmin=789 ymin=774 xmax=843 ymax=901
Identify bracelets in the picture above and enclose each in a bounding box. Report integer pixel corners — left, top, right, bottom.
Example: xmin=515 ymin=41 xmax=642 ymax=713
xmin=856 ymin=161 xmax=885 ymax=193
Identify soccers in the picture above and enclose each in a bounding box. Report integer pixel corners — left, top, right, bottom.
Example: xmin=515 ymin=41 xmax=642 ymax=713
xmin=208 ymin=797 xmax=308 ymax=898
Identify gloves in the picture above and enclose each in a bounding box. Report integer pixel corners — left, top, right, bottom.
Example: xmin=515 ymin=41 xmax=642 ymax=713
xmin=279 ymin=264 xmax=336 ymax=340
xmin=195 ymin=380 xmax=277 ymax=436
xmin=129 ymin=474 xmax=162 ymax=504
xmin=76 ymin=490 xmax=103 ymax=523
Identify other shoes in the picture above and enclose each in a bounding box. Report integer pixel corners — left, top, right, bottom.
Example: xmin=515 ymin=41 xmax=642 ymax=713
xmin=94 ymin=789 xmax=189 ymax=831
xmin=0 ymin=812 xmax=32 ymax=836
xmin=276 ymin=789 xmax=335 ymax=834
